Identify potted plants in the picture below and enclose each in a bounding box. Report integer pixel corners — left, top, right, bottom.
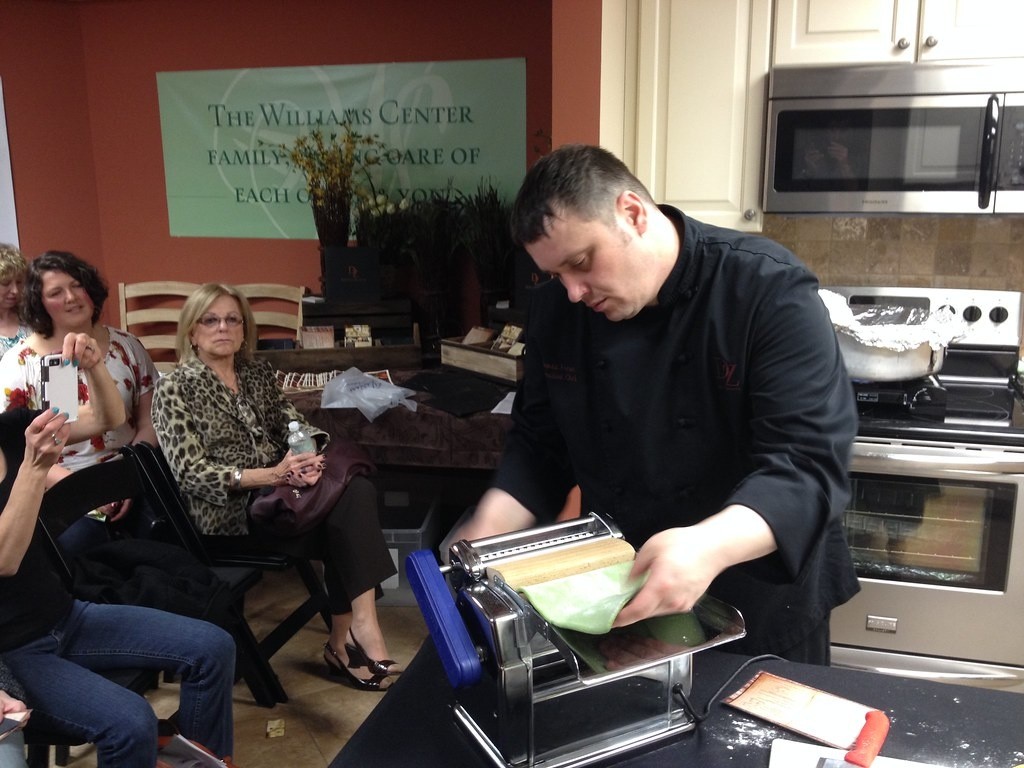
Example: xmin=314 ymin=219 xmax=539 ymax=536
xmin=397 ymin=179 xmax=519 ymax=352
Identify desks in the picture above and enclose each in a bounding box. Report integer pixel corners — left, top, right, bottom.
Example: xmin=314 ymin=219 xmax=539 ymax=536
xmin=289 ymin=369 xmax=514 ymax=469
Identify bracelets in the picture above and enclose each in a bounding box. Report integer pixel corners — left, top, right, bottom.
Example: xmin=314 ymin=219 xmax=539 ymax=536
xmin=234 ymin=468 xmax=243 ymax=490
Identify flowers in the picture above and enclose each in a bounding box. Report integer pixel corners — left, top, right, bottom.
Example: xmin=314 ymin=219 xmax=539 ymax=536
xmin=291 ymin=124 xmax=410 ymax=259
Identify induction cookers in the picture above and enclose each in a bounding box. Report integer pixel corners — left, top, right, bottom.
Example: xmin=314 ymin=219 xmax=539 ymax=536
xmin=818 ymin=286 xmax=1024 ymax=447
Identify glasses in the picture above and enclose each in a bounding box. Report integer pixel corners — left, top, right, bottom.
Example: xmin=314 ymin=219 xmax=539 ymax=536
xmin=197 ymin=316 xmax=245 ymax=327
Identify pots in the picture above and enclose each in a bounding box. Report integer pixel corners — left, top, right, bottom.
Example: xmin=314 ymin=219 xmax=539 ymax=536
xmin=835 ymin=329 xmax=944 ymax=381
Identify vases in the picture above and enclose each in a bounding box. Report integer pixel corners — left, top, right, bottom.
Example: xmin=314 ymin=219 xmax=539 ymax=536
xmin=317 ymin=247 xmax=405 ymax=297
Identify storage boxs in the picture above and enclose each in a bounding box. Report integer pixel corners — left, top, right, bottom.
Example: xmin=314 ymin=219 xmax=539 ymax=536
xmin=352 ymin=472 xmax=439 ymax=609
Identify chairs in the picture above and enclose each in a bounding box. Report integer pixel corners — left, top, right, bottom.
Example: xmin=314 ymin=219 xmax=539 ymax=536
xmin=2 ymin=278 xmax=331 ymax=768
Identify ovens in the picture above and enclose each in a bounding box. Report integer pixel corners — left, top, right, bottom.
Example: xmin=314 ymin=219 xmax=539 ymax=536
xmin=829 ymin=436 xmax=1024 ymax=694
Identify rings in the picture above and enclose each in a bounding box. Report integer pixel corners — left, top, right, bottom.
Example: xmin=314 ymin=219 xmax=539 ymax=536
xmin=53 ymin=436 xmax=62 ymax=445
xmin=50 ymin=432 xmax=57 ymax=441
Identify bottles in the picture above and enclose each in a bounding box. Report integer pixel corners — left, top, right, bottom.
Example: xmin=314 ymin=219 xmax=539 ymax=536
xmin=288 ymin=421 xmax=317 ymax=457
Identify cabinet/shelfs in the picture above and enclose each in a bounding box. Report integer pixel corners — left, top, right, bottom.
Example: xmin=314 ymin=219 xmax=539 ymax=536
xmin=626 ymin=1 xmax=1024 ymax=233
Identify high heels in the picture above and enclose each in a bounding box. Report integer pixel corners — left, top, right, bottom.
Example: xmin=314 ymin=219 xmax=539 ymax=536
xmin=323 ymin=625 xmax=405 ymax=691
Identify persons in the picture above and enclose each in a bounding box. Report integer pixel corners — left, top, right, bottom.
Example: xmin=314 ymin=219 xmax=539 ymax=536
xmin=439 ymin=144 xmax=862 ymax=667
xmin=151 ymin=285 xmax=405 ymax=692
xmin=0 ymin=243 xmax=236 ymax=768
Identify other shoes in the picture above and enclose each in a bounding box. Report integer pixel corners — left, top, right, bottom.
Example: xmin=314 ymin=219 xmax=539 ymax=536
xmin=224 ymin=756 xmax=237 ymax=768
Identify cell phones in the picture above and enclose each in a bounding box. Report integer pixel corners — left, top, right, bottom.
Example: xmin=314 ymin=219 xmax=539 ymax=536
xmin=41 ymin=354 xmax=79 ymax=423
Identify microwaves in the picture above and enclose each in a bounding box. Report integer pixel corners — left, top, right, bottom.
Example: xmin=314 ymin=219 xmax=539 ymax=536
xmin=762 ymin=66 xmax=1024 ymax=213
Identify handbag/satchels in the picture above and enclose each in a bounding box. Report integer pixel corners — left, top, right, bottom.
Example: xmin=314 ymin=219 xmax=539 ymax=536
xmin=156 ymin=719 xmax=223 ymax=768
xmin=320 ymin=367 xmax=418 ymax=424
xmin=250 ymin=438 xmax=376 ymax=529
